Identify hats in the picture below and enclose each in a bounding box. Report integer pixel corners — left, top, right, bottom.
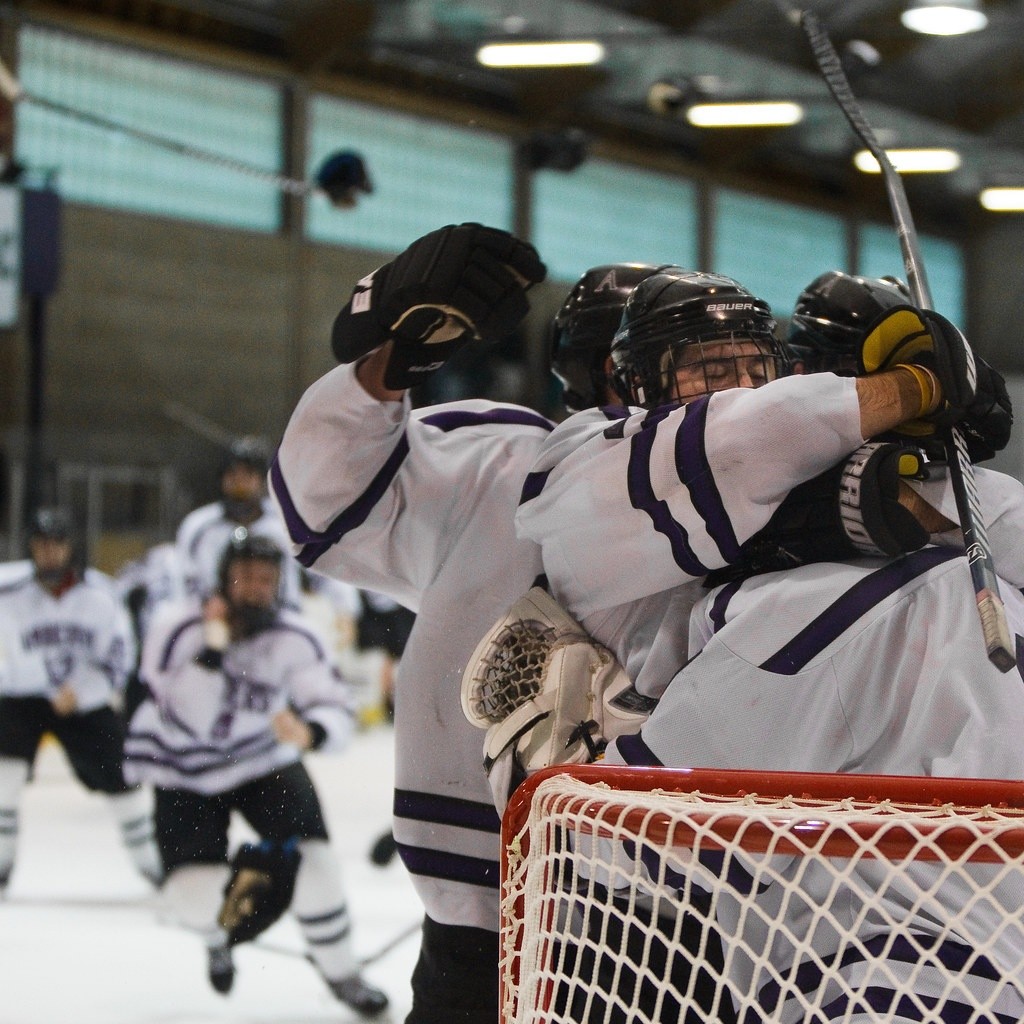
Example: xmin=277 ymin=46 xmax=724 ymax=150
xmin=220 ymin=537 xmax=284 ymax=566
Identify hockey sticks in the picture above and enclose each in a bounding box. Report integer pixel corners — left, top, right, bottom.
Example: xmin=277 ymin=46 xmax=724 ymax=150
xmin=801 ymin=12 xmax=1019 ymax=673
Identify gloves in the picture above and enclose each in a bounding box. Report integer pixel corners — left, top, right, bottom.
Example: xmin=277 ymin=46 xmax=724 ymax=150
xmin=857 ymin=307 xmax=1015 ymax=464
xmin=326 ymin=222 xmax=549 ymax=393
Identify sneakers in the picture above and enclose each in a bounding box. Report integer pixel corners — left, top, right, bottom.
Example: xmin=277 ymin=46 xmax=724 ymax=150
xmin=324 ymin=972 xmax=384 ymax=1016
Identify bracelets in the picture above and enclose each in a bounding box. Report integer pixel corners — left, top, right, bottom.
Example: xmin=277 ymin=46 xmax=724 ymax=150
xmin=895 ymin=362 xmax=942 ymax=415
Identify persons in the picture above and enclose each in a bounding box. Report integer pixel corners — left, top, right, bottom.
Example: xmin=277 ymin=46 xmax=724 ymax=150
xmin=119 ymin=534 xmax=390 ymax=1024
xmin=172 ymin=430 xmax=309 ymax=589
xmin=552 ymin=271 xmax=1024 ymax=1024
xmin=0 ymin=503 xmax=168 ymax=895
xmin=523 ymin=263 xmax=1014 ymax=1024
xmin=269 ymin=222 xmax=658 ymax=1024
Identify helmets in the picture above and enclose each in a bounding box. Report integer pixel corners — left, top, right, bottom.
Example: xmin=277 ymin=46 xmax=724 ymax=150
xmin=554 ymin=264 xmax=777 ymax=405
xmin=786 ymin=273 xmax=909 ymax=367
xmin=218 ymin=435 xmax=271 ymax=471
xmin=21 ymin=505 xmax=88 ymax=550
